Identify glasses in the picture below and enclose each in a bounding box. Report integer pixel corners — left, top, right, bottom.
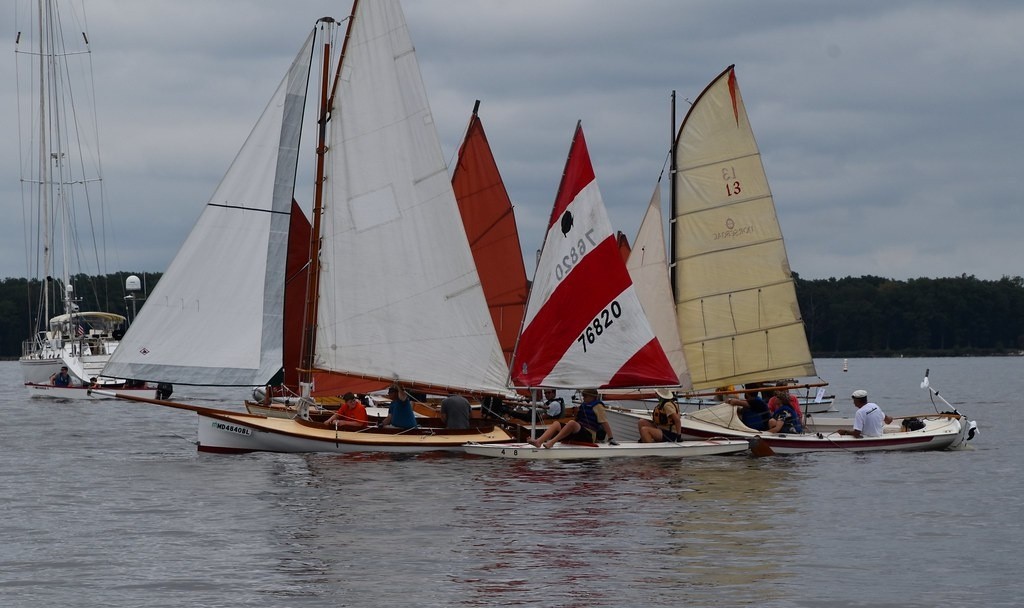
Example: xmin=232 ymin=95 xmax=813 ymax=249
xmin=61 ymin=369 xmax=65 ymax=371
xmin=544 ymin=391 xmax=551 ymax=395
xmin=347 ymin=399 xmax=355 ymax=403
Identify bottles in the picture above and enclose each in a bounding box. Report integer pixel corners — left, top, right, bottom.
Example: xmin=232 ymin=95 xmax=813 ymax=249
xmin=378 ymin=413 xmax=383 ymax=429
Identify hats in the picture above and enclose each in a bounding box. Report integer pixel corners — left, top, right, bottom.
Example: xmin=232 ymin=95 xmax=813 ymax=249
xmin=582 ymin=389 xmax=599 ymax=396
xmin=388 ymin=388 xmax=398 ymax=394
xmin=851 ymin=390 xmax=868 ymax=398
xmin=654 ymin=388 xmax=673 ymax=399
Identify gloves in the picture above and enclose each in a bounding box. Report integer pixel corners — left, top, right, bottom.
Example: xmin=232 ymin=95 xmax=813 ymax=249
xmin=677 ymin=434 xmax=683 ymax=442
xmin=608 ymin=438 xmax=620 ymax=446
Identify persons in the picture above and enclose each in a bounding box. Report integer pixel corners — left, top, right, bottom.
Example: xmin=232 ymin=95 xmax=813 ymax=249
xmin=537 ymin=389 xmax=561 ymax=424
xmin=51 ymin=366 xmax=73 ymax=388
xmin=768 ymin=381 xmax=806 ymax=425
xmin=768 ymin=397 xmax=802 ymax=433
xmin=378 ymin=385 xmax=417 ymax=430
xmin=714 ymin=385 xmax=739 ymax=401
xmin=324 ymin=392 xmax=368 ymax=427
xmin=725 ymin=384 xmax=771 ymax=430
xmin=638 ymin=388 xmax=682 ymax=443
xmin=527 ymin=388 xmax=620 ymax=448
xmin=838 ymin=390 xmax=894 ymax=437
xmin=88 ymin=377 xmax=101 ymax=389
xmin=441 ymin=390 xmax=473 ymax=429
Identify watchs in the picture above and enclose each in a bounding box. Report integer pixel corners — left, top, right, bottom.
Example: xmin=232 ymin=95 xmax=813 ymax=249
xmin=728 ymin=399 xmax=732 ymax=404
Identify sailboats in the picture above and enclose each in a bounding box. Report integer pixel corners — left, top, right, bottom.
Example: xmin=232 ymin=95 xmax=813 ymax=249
xmin=92 ymin=0 xmax=531 ymax=456
xmin=606 ymin=65 xmax=980 ymax=456
xmin=451 ymin=97 xmax=750 ymax=461
xmin=16 ymin=0 xmax=838 ymax=425
